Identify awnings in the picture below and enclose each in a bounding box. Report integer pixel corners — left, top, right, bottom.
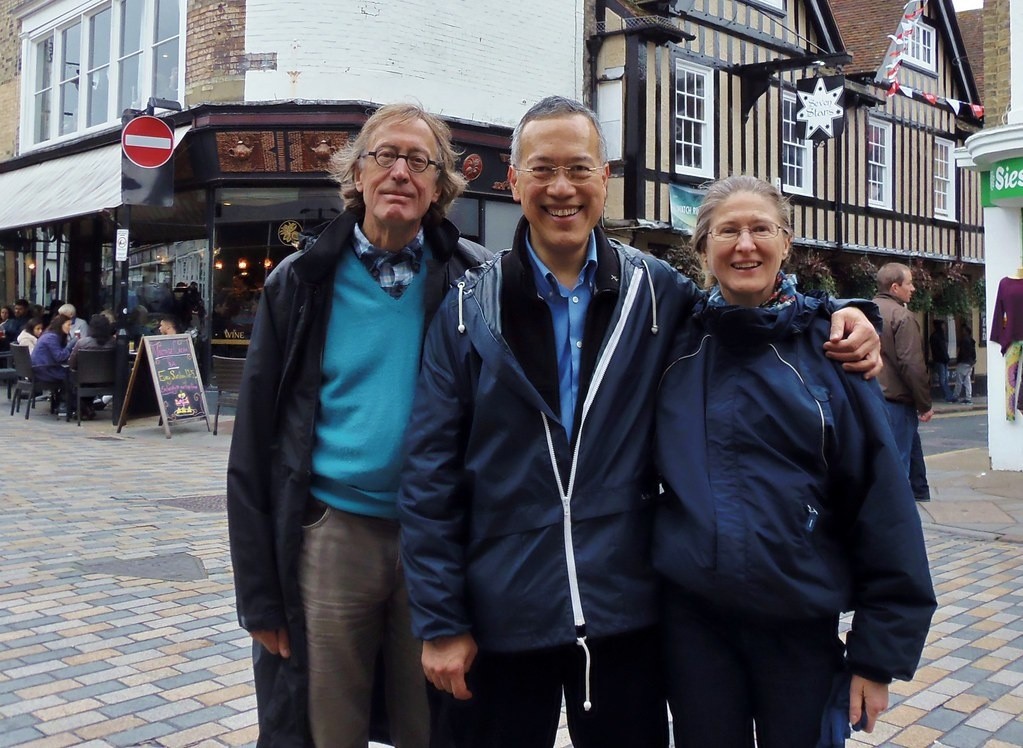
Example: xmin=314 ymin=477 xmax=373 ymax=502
xmin=0 ymin=125 xmax=192 ymax=229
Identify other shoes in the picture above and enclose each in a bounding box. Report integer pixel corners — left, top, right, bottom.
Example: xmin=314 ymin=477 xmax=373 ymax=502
xmin=56 ymin=399 xmax=106 ymax=419
xmin=960 ymin=400 xmax=972 ymax=405
xmin=946 ymin=397 xmax=958 ymax=403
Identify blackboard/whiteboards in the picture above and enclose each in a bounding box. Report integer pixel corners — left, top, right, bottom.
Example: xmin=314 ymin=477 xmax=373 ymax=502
xmin=118 ymin=331 xmax=210 ymax=426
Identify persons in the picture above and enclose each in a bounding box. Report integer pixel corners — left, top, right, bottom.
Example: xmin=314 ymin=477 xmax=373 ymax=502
xmin=952 ymin=324 xmax=976 ymax=403
xmin=17 ymin=317 xmax=43 ymax=357
xmin=870 ymin=262 xmax=933 ymax=484
xmin=118 ymin=304 xmax=157 ymax=350
xmin=99 ymin=309 xmax=117 ymax=343
xmin=227 ymin=96 xmax=497 ymax=748
xmin=67 ymin=314 xmax=117 ymax=418
xmin=909 ymin=429 xmax=930 ymax=502
xmin=0 ymin=299 xmax=32 ymax=348
xmin=929 ymin=320 xmax=954 ymax=403
xmin=41 ymin=300 xmax=65 ymax=329
xmin=0 ymin=306 xmax=13 ymax=351
xmin=159 ymin=314 xmax=182 ymax=334
xmin=159 ymin=282 xmax=203 ymax=334
xmin=17 ymin=304 xmax=45 ymax=335
xmin=213 ymin=293 xmax=235 ymax=337
xmin=58 ymin=303 xmax=87 ymax=346
xmin=990 ymin=263 xmax=1023 ymax=422
xmin=644 ymin=168 xmax=939 ymax=748
xmin=31 ymin=313 xmax=76 ymax=416
xmin=399 ymin=95 xmax=885 ymax=748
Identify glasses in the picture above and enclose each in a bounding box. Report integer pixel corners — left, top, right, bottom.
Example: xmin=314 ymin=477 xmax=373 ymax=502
xmin=357 ymin=147 xmax=442 ymax=173
xmin=705 ymin=220 xmax=789 ymax=242
xmin=512 ymin=166 xmax=605 ymax=186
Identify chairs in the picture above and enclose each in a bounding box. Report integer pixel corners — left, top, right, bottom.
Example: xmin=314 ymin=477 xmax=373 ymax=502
xmin=0 ymin=336 xmax=248 ymax=435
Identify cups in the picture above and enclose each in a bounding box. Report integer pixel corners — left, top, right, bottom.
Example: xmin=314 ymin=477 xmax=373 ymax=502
xmin=75 ymin=330 xmax=81 ymax=339
xmin=129 ymin=340 xmax=134 ymax=350
